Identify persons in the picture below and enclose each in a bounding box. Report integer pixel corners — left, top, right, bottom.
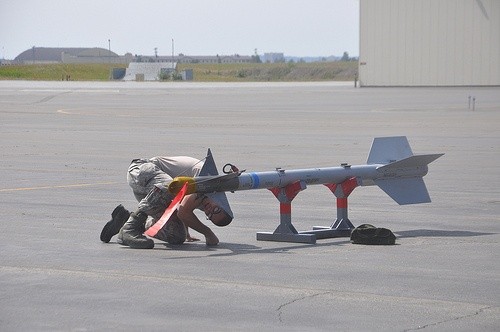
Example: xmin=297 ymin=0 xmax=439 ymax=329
xmin=99 ymin=155 xmax=232 ymax=249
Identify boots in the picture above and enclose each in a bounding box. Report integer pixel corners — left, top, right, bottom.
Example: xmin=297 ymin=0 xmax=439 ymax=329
xmin=100 ymin=204 xmax=130 ymax=243
xmin=117 ymin=212 xmax=154 ymax=249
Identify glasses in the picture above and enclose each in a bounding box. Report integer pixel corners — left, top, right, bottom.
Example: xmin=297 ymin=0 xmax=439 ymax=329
xmin=206 ymin=207 xmax=223 ymax=220
